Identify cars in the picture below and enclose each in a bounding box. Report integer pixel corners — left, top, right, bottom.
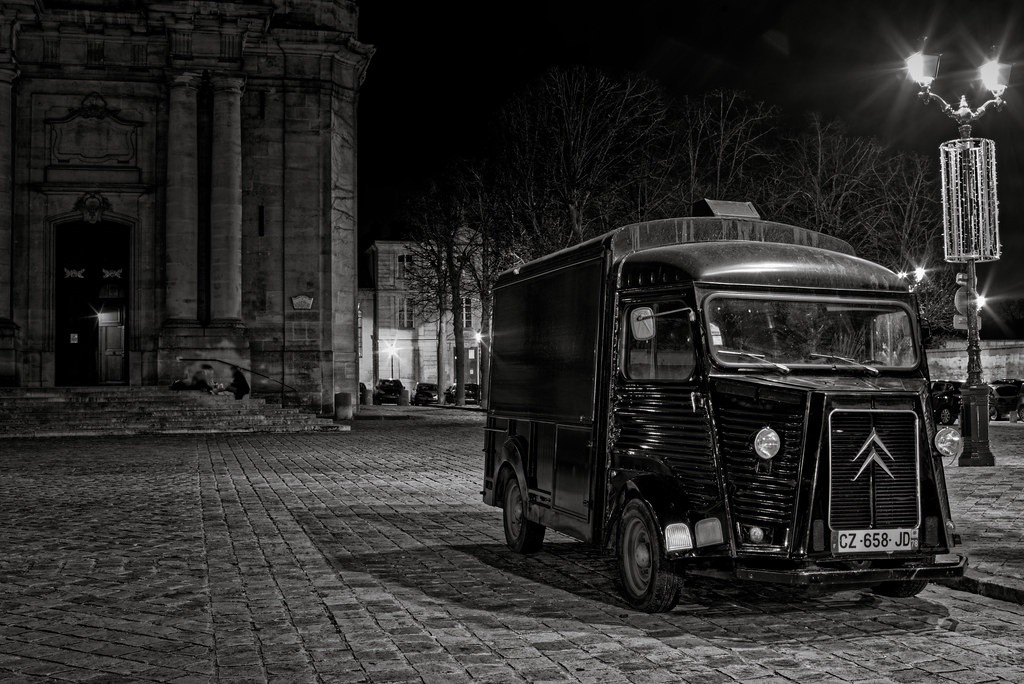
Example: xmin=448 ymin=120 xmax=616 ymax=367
xmin=410 ymin=382 xmax=441 ymax=407
xmin=927 ymin=379 xmax=999 ymax=426
xmin=445 ymin=382 xmax=481 ymax=405
xmin=372 ymin=378 xmax=405 ymax=406
xmin=986 ymin=377 xmax=1024 ymax=425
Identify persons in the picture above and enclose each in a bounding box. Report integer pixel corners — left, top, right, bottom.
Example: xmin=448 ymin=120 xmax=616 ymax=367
xmin=193 ymin=369 xmax=215 ymax=394
xmin=225 ymin=366 xmax=251 ymax=400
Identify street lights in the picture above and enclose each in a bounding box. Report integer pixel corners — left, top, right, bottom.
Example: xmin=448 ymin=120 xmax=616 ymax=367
xmin=905 ymin=50 xmax=1002 ymax=469
xmin=388 ymin=343 xmax=395 ymax=380
xmin=475 ymin=332 xmax=480 ymax=405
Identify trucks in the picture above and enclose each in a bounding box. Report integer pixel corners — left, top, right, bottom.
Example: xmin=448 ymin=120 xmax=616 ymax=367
xmin=480 ymin=195 xmax=967 ymax=617
